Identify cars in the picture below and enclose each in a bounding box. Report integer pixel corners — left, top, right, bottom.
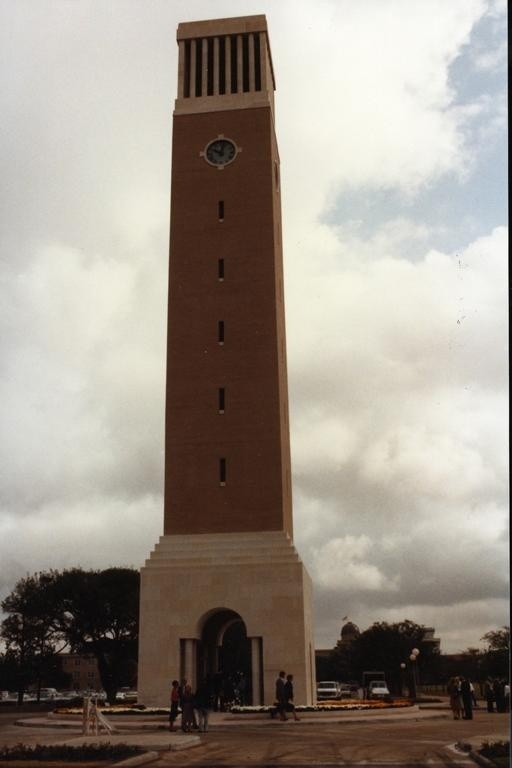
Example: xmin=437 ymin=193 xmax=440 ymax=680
xmin=1 ymin=686 xmax=137 ymax=702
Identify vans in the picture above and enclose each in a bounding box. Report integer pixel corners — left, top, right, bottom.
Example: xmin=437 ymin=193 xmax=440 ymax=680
xmin=316 ymin=671 xmax=390 ymax=700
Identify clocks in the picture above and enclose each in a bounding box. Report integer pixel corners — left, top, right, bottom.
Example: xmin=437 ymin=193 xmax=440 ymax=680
xmin=199 ymin=133 xmax=243 ymax=171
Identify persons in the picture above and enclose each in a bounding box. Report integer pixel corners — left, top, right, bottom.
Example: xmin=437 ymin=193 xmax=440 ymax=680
xmin=169 ymin=679 xmax=209 ymax=733
xmin=285 ymin=675 xmax=300 ymax=721
xmin=276 ymin=672 xmax=286 ymax=721
xmin=447 ymin=674 xmax=510 ymax=720
